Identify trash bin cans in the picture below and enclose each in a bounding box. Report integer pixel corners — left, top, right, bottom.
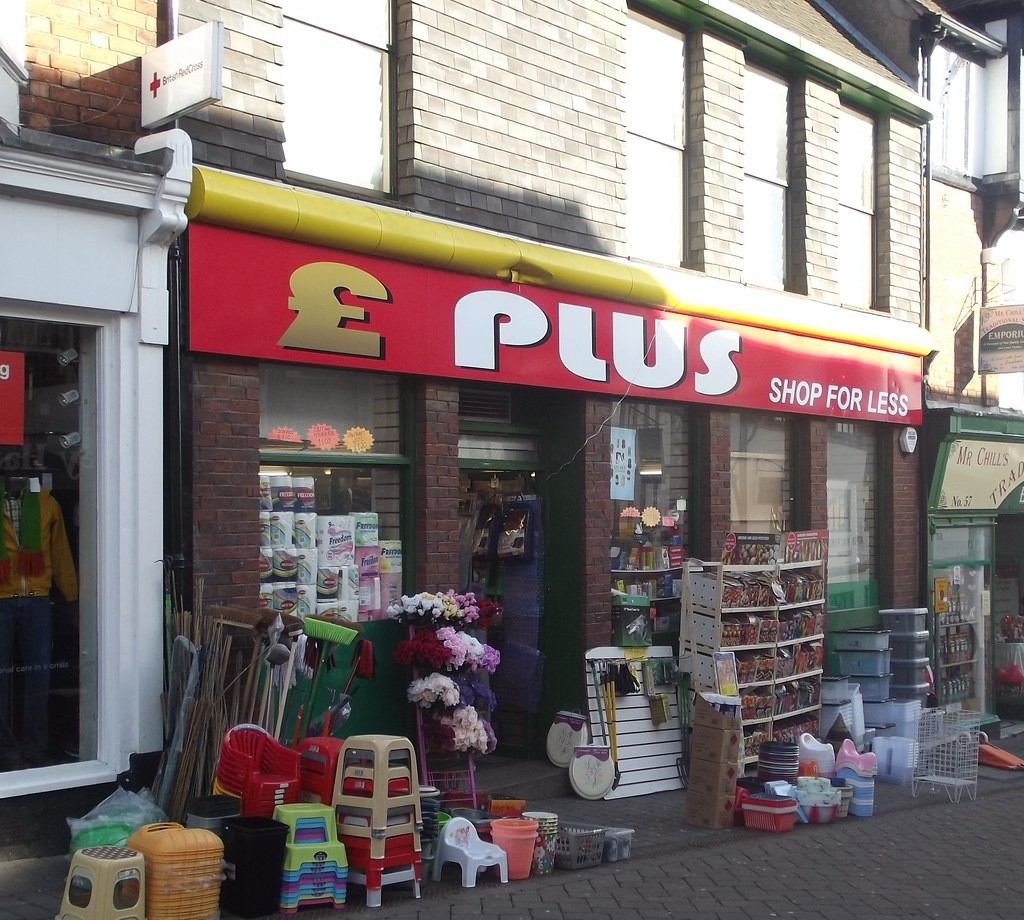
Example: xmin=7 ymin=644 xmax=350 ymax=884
xmin=756 ymin=740 xmax=800 ymax=785
xmin=490 ymin=812 xmax=558 ymax=880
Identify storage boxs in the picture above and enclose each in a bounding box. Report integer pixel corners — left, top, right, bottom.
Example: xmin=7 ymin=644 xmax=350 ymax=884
xmin=879 ymin=631 xmax=930 ymax=659
xmin=830 ymin=630 xmax=891 ymax=651
xmin=890 ymin=657 xmax=929 ymax=686
xmin=736 ymin=776 xmax=769 ymax=793
xmin=602 ymin=828 xmax=636 ymax=861
xmin=221 ymin=815 xmax=290 ymax=917
xmin=691 ymin=571 xmax=818 ymax=762
xmin=687 ymin=760 xmax=739 ymax=796
xmin=878 ymin=607 xmax=928 ymax=632
xmin=833 ymin=648 xmax=893 ymax=674
xmin=616 ymin=594 xmax=652 ymax=645
xmin=821 ymin=676 xmax=929 ymax=785
xmin=684 ymin=789 xmax=735 ymax=828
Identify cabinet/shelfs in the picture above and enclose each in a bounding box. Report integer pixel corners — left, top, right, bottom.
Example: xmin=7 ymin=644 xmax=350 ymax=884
xmin=676 ymin=556 xmax=825 ymax=764
xmin=615 ymin=567 xmax=683 ymax=643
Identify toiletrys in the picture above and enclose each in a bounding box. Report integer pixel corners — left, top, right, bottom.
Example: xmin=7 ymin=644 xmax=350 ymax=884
xmin=634 ymin=578 xmax=654 ymax=599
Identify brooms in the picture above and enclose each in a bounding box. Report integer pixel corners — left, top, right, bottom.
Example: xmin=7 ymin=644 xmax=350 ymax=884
xmin=207 ymin=603 xmax=364 ymax=758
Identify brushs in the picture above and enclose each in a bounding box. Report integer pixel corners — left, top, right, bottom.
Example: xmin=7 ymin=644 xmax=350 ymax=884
xmin=205 ymin=603 xmax=372 ymax=749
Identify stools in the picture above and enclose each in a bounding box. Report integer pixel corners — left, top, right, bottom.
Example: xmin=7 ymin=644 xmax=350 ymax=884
xmin=53 ymin=847 xmax=147 ymax=920
xmin=272 ymin=734 xmax=423 ymax=913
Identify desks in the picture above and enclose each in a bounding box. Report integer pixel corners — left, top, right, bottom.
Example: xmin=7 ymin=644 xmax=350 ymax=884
xmin=995 ymin=643 xmax=1024 ymax=697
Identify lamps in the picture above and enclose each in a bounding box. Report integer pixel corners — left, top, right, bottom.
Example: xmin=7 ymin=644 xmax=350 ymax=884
xmin=57 ymin=349 xmax=84 ymax=366
xmin=55 ymin=389 xmax=82 ymax=408
xmin=59 ymin=431 xmax=84 ymax=450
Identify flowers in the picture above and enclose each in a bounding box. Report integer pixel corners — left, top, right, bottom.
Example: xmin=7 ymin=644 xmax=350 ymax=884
xmin=388 ymin=588 xmax=499 ymax=629
xmin=406 ymin=672 xmax=459 ymax=710
xmin=455 ymin=679 xmax=497 ymax=711
xmin=393 ymin=626 xmax=504 ymax=674
xmin=422 ymin=704 xmax=499 ymax=754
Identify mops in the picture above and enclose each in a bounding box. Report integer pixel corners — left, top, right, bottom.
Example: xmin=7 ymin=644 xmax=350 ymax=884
xmin=344 ymin=638 xmax=380 ymax=695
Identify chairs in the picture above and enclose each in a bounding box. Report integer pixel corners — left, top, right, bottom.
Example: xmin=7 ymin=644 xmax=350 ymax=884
xmin=212 ymin=722 xmax=303 ymax=823
xmin=431 ymin=815 xmax=510 ymax=888
